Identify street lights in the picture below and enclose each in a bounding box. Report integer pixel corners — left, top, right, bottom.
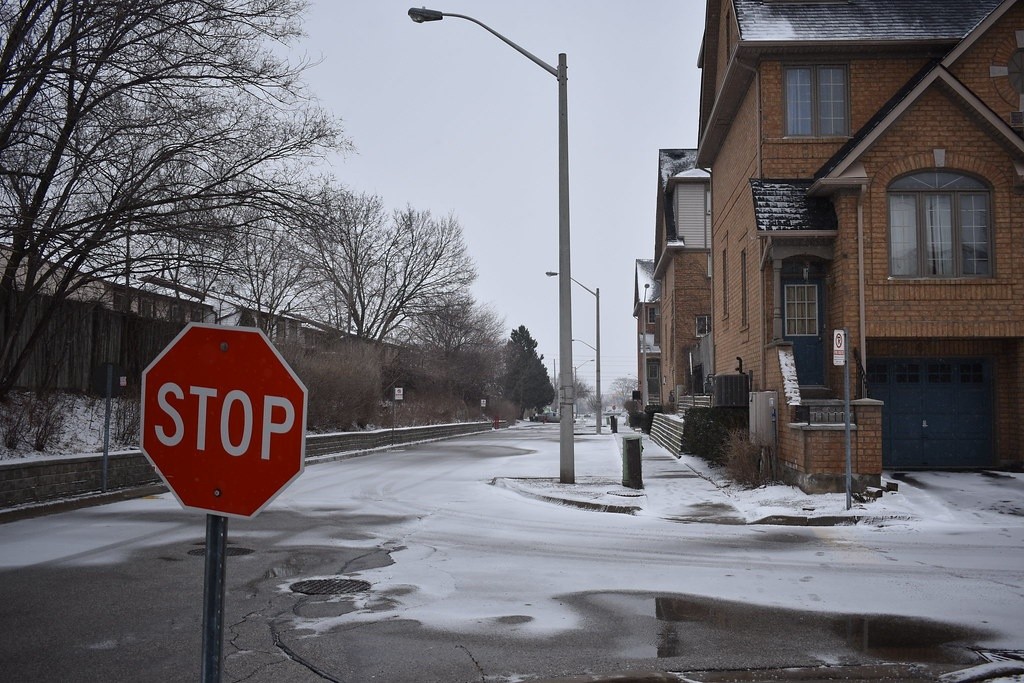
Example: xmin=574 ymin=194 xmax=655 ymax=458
xmin=544 ymin=271 xmax=602 ymax=435
xmin=407 ymin=5 xmax=576 ymax=485
xmin=575 ymin=359 xmax=595 ymax=419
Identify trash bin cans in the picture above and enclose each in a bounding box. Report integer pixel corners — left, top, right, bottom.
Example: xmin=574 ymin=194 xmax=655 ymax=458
xmin=605 ymin=416 xmax=618 ymax=434
xmin=622 ymin=436 xmax=645 ymax=490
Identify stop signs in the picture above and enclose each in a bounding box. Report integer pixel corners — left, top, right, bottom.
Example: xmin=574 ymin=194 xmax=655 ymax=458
xmin=138 ymin=323 xmax=308 ymax=521
